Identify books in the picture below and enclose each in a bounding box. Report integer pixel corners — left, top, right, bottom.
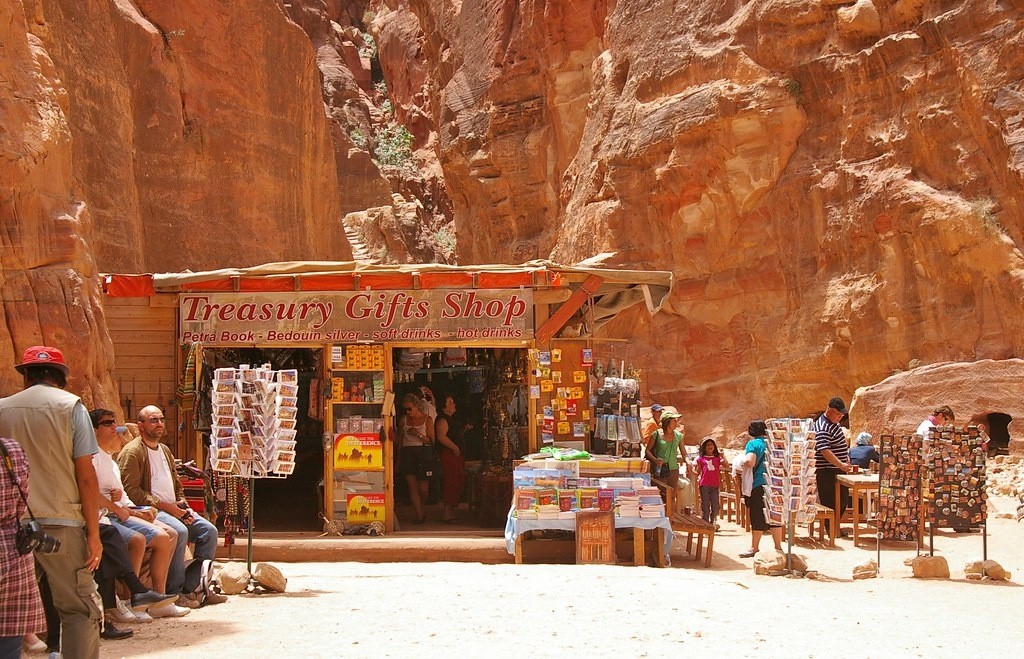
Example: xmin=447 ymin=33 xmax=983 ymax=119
xmin=512 ymin=472 xmax=663 ymax=519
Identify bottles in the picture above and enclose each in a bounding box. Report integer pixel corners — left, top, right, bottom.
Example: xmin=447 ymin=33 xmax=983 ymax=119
xmin=870 ymin=460 xmax=873 ymax=473
xmin=344 ymin=379 xmax=370 ymax=402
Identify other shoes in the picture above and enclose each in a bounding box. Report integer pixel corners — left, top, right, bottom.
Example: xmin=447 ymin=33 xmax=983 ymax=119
xmin=412 ymin=516 xmax=424 ymax=524
xmin=22 ymin=634 xmax=49 ymax=653
xmin=443 ymin=515 xmax=462 ymax=523
xmin=740 ymin=550 xmax=759 ymax=558
xmin=840 ymin=531 xmax=848 ymax=536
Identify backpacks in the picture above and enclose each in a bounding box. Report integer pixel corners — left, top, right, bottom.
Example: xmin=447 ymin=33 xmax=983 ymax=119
xmin=184 ymin=558 xmax=214 ymax=606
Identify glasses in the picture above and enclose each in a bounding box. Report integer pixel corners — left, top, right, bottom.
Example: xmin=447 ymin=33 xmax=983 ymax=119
xmin=943 ymin=413 xmax=950 ymax=423
xmin=96 ymin=419 xmax=116 ymax=427
xmin=402 ymin=405 xmax=416 ymax=412
xmin=140 ymin=417 xmax=166 ymax=424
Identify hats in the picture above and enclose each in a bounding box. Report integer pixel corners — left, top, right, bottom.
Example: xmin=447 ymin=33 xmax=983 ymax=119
xmin=660 ymin=412 xmax=682 ymax=420
xmin=14 ymin=346 xmax=70 ymax=377
xmin=651 ymin=404 xmax=664 ymax=412
xmin=701 ymin=436 xmax=716 ymax=447
xmin=829 ymin=398 xmax=848 ymax=414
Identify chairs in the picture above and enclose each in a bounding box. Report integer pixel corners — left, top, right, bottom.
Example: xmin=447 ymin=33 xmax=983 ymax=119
xmin=718 ymin=467 xmax=835 ymax=548
xmin=651 ymin=479 xmax=716 ymax=568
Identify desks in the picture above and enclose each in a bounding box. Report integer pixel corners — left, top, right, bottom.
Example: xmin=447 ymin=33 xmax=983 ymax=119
xmin=835 ymin=473 xmax=925 ymax=548
xmin=504 ymin=503 xmax=673 ymax=565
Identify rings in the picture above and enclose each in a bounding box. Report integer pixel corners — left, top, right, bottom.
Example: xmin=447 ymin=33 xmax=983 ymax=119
xmin=95 ymin=556 xmax=99 ymax=561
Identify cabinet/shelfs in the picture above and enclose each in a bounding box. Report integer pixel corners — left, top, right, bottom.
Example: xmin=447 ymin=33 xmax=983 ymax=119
xmin=499 ymin=381 xmax=528 ymax=432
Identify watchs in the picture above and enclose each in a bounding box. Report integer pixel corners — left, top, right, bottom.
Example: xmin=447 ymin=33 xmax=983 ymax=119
xmin=418 ymin=434 xmax=424 ymax=439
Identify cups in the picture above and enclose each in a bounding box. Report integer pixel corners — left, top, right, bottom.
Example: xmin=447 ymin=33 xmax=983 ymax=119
xmin=853 ymin=465 xmax=859 ymax=474
xmin=863 ymin=469 xmax=871 ymax=476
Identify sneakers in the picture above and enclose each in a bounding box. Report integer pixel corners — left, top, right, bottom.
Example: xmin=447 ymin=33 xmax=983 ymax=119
xmin=148 ymin=601 xmax=190 ymax=618
xmin=122 ymin=600 xmax=152 ymax=623
xmin=204 ymin=589 xmax=227 ymax=604
xmin=173 ymin=594 xmax=198 ymax=607
xmin=105 ymin=601 xmax=136 ymax=622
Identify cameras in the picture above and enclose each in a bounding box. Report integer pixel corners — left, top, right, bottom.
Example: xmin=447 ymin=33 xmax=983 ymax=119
xmin=178 ymin=502 xmax=191 ymax=518
xmin=17 ymin=521 xmax=62 ymax=555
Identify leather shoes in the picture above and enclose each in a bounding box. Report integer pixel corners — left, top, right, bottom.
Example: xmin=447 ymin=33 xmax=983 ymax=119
xmin=100 ymin=620 xmax=134 ymax=639
xmin=131 ymin=589 xmax=180 ymax=611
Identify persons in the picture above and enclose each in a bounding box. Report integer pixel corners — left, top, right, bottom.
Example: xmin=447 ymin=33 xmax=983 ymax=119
xmin=844 ymin=431 xmax=879 ymax=472
xmin=80 ymin=522 xmax=180 ymax=640
xmin=645 ymin=405 xmax=691 ymax=514
xmin=741 ymin=418 xmax=784 ymax=559
xmin=85 ymin=407 xmax=191 ymax=617
xmin=916 ymin=405 xmax=955 ymax=464
xmin=394 ymin=386 xmax=439 ymax=525
xmin=815 ymin=396 xmax=854 ymax=537
xmin=0 ymin=347 xmax=103 ymax=659
xmin=642 ymin=403 xmax=663 ymax=448
xmin=692 ymin=436 xmax=729 ymax=531
xmin=433 ymin=394 xmax=473 ymax=523
xmin=117 ymin=405 xmax=228 ymax=609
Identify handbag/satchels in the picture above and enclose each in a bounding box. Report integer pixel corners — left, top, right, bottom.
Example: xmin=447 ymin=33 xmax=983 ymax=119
xmin=653 ymin=462 xmax=671 ymax=478
xmin=414 ymin=438 xmax=439 ymax=480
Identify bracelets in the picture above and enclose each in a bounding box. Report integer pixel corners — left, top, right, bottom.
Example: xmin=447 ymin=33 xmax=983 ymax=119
xmin=448 ymin=443 xmax=454 ymax=448
xmin=133 ymin=510 xmax=136 ymax=516
